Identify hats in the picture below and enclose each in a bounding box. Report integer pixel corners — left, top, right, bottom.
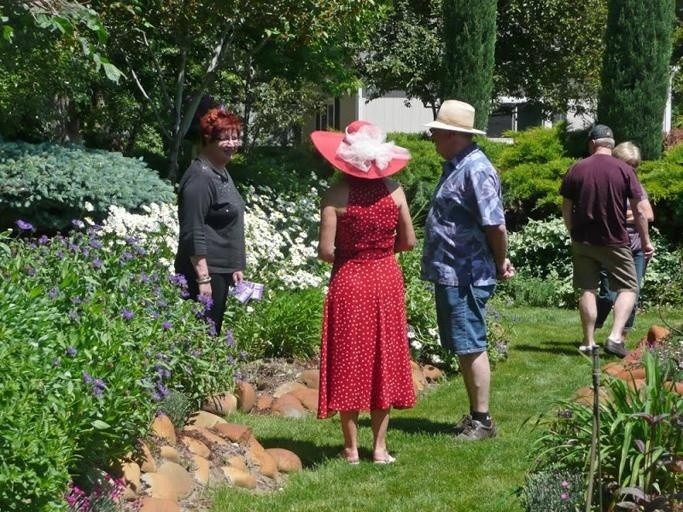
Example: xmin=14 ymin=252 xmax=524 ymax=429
xmin=423 ymin=100 xmax=486 ymax=136
xmin=583 ymin=124 xmax=615 ymax=143
xmin=310 ymin=120 xmax=409 ymax=180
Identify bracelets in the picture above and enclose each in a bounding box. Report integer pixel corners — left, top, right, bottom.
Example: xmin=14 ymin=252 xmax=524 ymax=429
xmin=196 ymin=276 xmax=213 ymax=284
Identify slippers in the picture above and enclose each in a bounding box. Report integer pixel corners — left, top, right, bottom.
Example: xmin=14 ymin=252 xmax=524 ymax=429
xmin=373 ymin=454 xmax=396 ymax=465
xmin=338 ymin=453 xmax=360 ymax=465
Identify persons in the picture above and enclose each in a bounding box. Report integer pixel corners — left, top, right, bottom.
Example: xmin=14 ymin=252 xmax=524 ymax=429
xmin=318 ymin=172 xmax=416 ymax=464
xmin=559 ymin=125 xmax=656 ymax=359
xmin=420 ymin=99 xmax=517 ymax=445
xmin=176 ymin=107 xmax=250 ymax=337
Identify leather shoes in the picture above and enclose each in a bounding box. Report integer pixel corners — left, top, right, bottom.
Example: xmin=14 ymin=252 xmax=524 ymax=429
xmin=575 ymin=344 xmax=600 ymax=358
xmin=444 ymin=415 xmax=496 ymax=441
xmin=605 ymin=340 xmax=629 ymax=358
